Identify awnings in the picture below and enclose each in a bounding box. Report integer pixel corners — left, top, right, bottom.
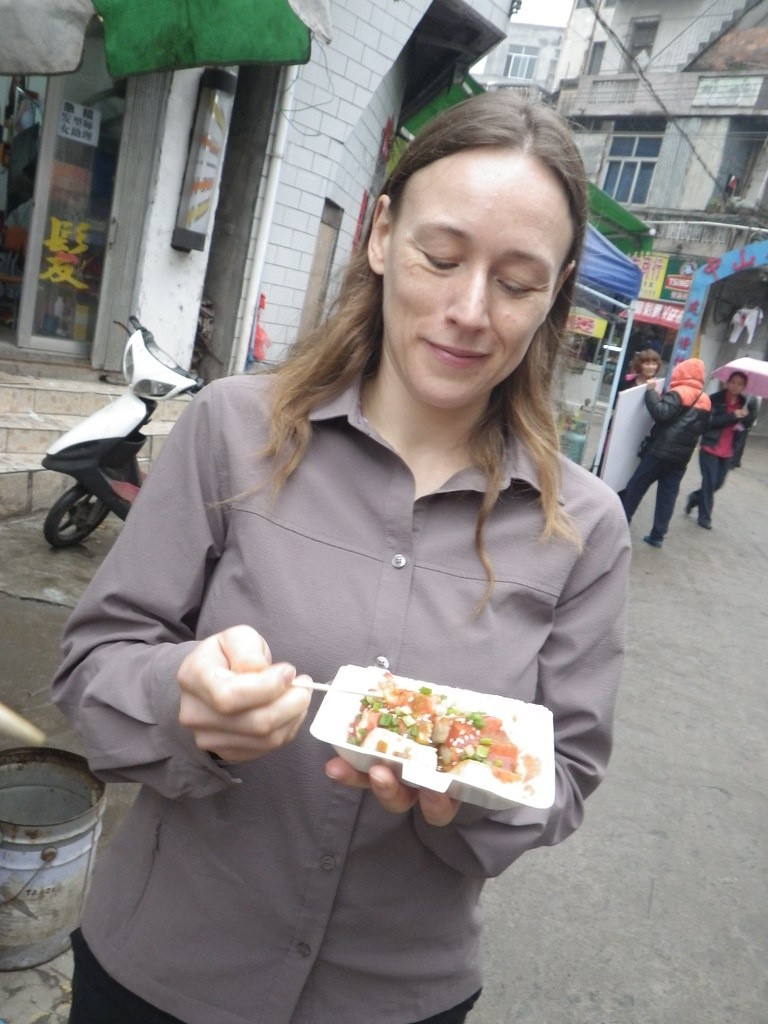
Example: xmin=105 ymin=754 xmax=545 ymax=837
xmin=399 ymin=72 xmax=654 ymax=257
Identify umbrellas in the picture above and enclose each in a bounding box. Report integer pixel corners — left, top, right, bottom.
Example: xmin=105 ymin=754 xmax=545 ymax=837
xmin=0 ymin=0 xmax=332 ymax=83
xmin=709 ymin=357 xmax=768 ymax=397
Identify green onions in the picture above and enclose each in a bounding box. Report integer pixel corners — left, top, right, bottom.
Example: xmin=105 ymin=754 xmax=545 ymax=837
xmin=349 ymin=685 xmax=504 ymax=767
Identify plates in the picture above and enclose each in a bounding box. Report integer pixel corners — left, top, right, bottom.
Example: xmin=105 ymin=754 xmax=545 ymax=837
xmin=308 ymin=663 xmax=557 ymax=812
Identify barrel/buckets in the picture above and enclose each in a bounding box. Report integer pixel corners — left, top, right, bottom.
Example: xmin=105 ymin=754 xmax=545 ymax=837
xmin=0 ymin=746 xmax=107 ymax=973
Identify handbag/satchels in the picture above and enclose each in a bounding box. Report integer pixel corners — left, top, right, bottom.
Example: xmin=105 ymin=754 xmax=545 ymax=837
xmin=636 ymin=440 xmax=650 ymax=457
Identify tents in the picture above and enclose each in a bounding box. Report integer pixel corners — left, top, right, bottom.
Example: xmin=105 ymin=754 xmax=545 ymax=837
xmin=576 ymin=222 xmax=643 ymax=474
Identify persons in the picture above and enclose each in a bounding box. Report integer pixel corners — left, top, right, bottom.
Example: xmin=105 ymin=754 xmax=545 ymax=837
xmin=591 ymin=350 xmax=662 ymax=476
xmin=687 ymin=371 xmax=758 ymax=529
xmin=45 ymin=92 xmax=636 ymax=1024
xmin=617 ymin=357 xmax=711 ymax=547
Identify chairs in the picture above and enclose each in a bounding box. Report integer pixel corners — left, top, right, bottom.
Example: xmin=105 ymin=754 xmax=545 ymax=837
xmin=0 ymin=225 xmax=26 ymax=330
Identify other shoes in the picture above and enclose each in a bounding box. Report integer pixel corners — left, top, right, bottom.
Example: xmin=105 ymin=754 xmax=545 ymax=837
xmin=685 ymin=494 xmax=696 ymax=515
xmin=644 ymin=534 xmax=662 ymax=548
xmin=696 ymin=519 xmax=711 ymax=529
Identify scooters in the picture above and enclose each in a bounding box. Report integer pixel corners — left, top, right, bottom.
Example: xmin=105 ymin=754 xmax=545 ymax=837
xmin=40 ymin=315 xmax=206 ymax=547
